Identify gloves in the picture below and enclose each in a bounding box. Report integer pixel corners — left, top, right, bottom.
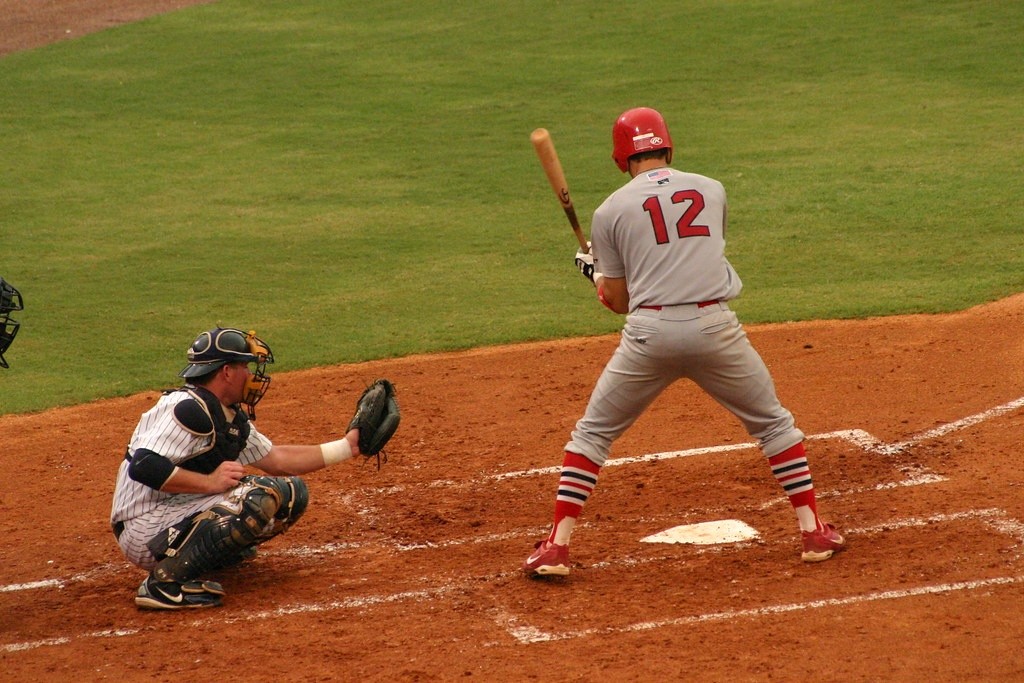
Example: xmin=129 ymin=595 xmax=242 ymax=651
xmin=574 ymin=241 xmax=603 ymax=286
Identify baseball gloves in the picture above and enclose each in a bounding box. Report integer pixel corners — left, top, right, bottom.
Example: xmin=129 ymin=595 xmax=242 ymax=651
xmin=345 ymin=378 xmax=400 ymax=473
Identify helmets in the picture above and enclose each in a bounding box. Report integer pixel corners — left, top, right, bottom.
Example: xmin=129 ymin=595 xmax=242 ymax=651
xmin=177 ymin=329 xmax=254 ymax=377
xmin=612 ymin=107 xmax=673 ymax=173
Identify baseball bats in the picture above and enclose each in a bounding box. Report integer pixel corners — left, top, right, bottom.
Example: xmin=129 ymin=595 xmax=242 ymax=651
xmin=531 ymin=127 xmax=589 ymax=254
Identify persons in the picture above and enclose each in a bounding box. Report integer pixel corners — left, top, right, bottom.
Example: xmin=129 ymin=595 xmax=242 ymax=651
xmin=521 ymin=105 xmax=844 ymax=577
xmin=109 ymin=326 xmax=362 ymax=610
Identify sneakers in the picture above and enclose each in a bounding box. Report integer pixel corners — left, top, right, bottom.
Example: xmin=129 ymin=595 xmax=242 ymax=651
xmin=135 ymin=575 xmax=215 ymax=608
xmin=523 ymin=541 xmax=570 ymax=576
xmin=801 ymin=522 xmax=846 ymax=562
